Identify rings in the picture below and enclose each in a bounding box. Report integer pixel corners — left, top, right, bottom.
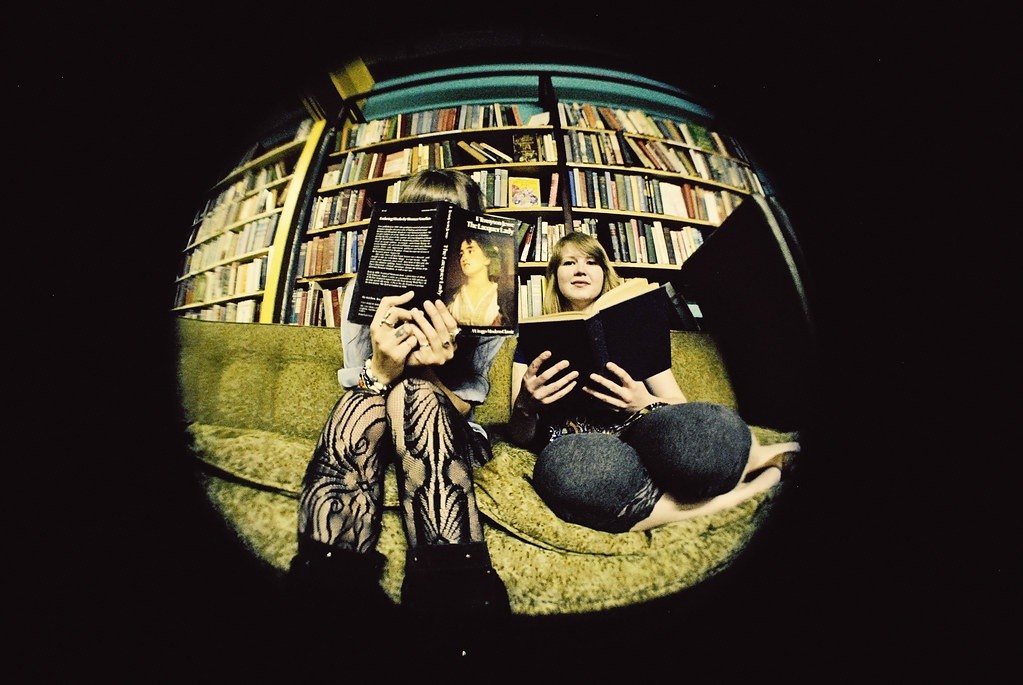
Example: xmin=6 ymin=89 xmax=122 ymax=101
xmin=442 ymin=342 xmax=450 ymax=348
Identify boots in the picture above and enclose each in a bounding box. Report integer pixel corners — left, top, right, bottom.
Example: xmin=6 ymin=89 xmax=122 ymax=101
xmin=276 ymin=533 xmax=395 ymax=671
xmin=399 ymin=546 xmax=513 ymax=667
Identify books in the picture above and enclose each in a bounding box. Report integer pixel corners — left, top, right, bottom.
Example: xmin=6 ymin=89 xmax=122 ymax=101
xmin=505 ymin=277 xmax=677 ymax=419
xmin=169 ymin=98 xmax=785 ymax=336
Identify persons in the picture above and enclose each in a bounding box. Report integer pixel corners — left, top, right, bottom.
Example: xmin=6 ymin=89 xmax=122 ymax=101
xmin=509 ymin=227 xmax=802 ymax=538
xmin=281 ymin=167 xmax=525 ymax=620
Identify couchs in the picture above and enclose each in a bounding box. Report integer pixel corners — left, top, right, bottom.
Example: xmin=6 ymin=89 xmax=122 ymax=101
xmin=162 ymin=319 xmax=799 ymax=616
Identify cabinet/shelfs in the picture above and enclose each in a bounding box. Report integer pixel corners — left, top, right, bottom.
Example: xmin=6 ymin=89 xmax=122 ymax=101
xmin=173 ymin=101 xmax=775 ymax=331
